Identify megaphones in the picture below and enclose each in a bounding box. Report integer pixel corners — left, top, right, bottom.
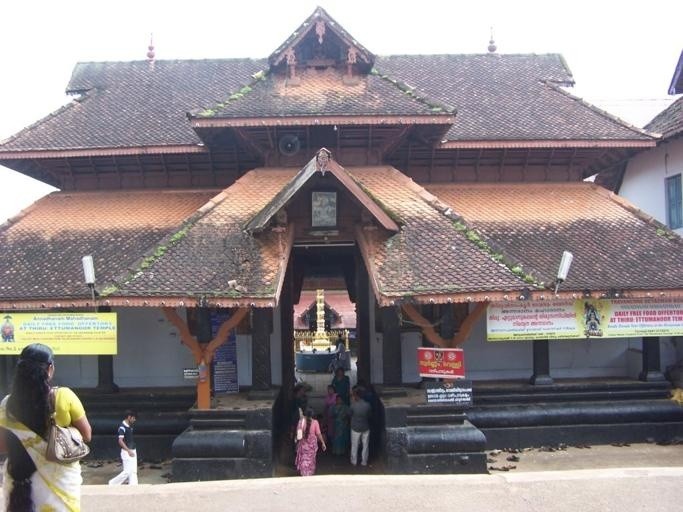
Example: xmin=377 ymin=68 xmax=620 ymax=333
xmin=279 ymin=134 xmax=300 ymax=156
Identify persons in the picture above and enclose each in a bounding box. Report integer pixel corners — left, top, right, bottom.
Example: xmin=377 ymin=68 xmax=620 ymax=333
xmin=0 ymin=343 xmax=92 ymax=512
xmin=583 ymin=302 xmax=599 ymax=327
xmin=108 ymin=409 xmax=139 ymax=483
xmin=288 ymin=334 xmax=374 ymax=476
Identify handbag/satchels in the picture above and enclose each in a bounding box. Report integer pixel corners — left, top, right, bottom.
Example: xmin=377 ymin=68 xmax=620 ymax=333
xmin=45 ymin=425 xmax=91 ymax=464
xmin=297 ymin=429 xmax=303 ymax=440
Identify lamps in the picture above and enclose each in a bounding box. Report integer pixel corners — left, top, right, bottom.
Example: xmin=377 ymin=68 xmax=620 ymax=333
xmin=81 ymin=255 xmax=96 ymax=301
xmin=554 ymin=251 xmax=573 ymax=294
